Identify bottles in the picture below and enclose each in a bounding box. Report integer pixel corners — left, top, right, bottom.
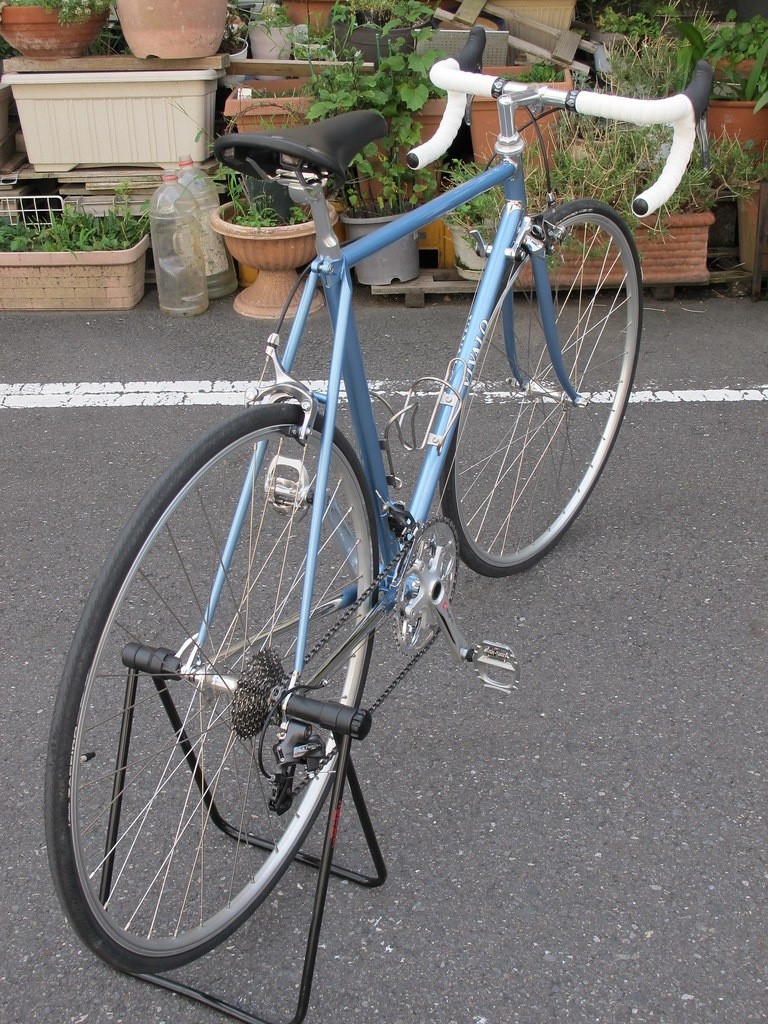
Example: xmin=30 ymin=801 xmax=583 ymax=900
xmin=177 ymin=155 xmax=238 ymax=299
xmin=148 ymin=169 xmax=209 ymax=319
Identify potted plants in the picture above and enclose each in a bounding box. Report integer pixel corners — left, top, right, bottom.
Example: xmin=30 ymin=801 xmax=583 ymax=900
xmin=0 ymin=0 xmax=767 ymax=320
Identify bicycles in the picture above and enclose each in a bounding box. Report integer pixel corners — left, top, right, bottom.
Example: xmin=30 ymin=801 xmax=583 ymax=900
xmin=43 ymin=26 xmax=715 ymax=1023
